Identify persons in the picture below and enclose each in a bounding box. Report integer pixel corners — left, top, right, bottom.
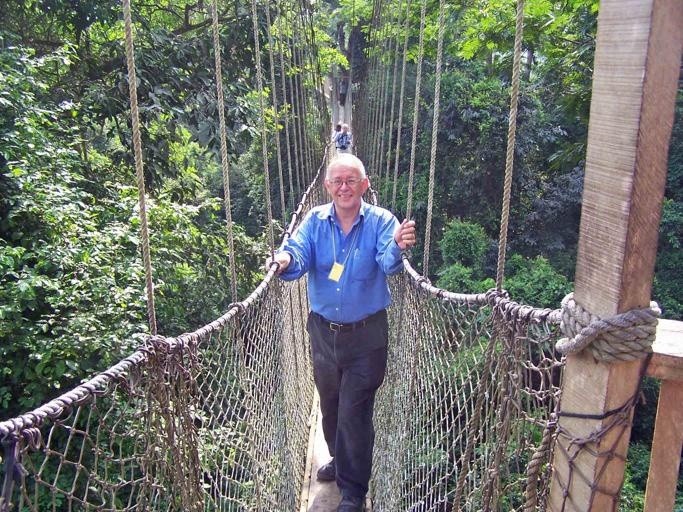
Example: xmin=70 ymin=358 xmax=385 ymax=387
xmin=338 ymin=79 xmax=348 ymax=107
xmin=330 ymin=124 xmax=341 ymax=139
xmin=325 ymin=123 xmax=354 ymax=154
xmin=263 ymin=151 xmax=417 ymax=512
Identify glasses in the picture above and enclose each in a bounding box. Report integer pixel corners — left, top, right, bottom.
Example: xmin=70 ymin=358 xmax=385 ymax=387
xmin=330 ymin=178 xmax=363 ymax=186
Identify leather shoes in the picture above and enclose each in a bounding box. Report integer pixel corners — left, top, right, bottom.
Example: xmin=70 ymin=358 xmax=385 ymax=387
xmin=317 ymin=457 xmax=336 ymax=481
xmin=337 ymin=495 xmax=366 ymax=512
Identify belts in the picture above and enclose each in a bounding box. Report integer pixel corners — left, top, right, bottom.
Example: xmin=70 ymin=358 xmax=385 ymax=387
xmin=320 ymin=315 xmax=376 ymax=332
